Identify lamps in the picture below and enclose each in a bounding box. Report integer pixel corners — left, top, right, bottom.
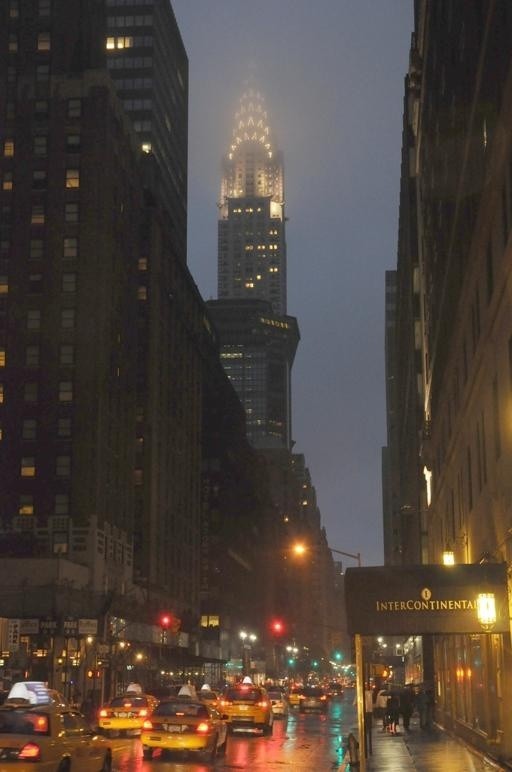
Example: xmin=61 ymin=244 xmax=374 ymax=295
xmin=476 ymin=592 xmax=512 ymax=633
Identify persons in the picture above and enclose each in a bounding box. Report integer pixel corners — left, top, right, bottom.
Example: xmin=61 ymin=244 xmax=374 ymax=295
xmin=372 ymin=679 xmax=437 ymax=736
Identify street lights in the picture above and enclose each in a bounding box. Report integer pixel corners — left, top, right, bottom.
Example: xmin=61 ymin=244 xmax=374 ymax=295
xmin=238 ymin=630 xmax=257 ymax=676
xmin=292 ymin=542 xmax=361 ymax=567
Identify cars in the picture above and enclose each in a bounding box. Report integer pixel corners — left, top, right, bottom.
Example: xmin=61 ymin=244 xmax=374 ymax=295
xmin=45 ymin=688 xmax=71 ymax=707
xmin=99 ymin=683 xmax=160 ymax=731
xmin=1 ymin=681 xmax=118 ymax=772
xmin=199 ymin=666 xmax=357 ymax=739
xmin=141 ymin=684 xmax=229 ymax=762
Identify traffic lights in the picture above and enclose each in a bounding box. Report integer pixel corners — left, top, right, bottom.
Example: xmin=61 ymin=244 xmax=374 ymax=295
xmin=161 ymin=614 xmax=171 ymax=631
